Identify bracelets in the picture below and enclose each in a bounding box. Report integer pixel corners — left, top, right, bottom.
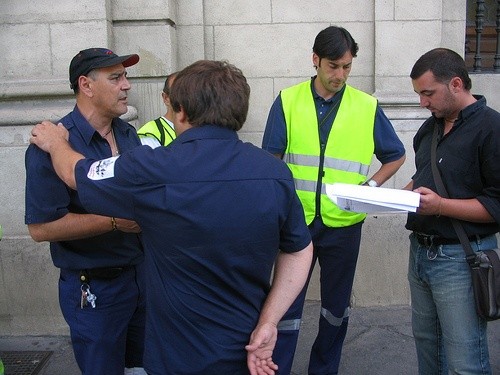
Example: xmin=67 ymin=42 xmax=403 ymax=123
xmin=111 ymin=216 xmax=121 ymax=232
xmin=436 ymin=197 xmax=443 ymax=218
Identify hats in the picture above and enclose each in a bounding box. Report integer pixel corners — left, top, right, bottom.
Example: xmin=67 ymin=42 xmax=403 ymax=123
xmin=68 ymin=48 xmax=139 ymax=89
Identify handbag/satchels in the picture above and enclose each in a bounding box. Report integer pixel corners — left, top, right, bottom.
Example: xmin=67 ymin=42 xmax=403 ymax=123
xmin=465 ymin=248 xmax=500 ymax=321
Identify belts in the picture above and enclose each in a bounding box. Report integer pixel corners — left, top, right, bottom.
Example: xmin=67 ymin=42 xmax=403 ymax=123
xmin=86 ymin=267 xmax=123 ymax=279
xmin=412 ymin=231 xmax=494 ymax=249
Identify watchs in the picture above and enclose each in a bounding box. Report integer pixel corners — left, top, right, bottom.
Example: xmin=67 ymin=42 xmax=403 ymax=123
xmin=366 ymin=178 xmax=378 ymax=187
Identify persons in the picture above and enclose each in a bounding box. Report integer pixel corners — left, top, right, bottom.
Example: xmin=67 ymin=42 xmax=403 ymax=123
xmin=262 ymin=26 xmax=405 ymax=375
xmin=30 ymin=60 xmax=313 ymax=375
xmin=401 ymin=48 xmax=500 ymax=375
xmin=25 ymin=48 xmax=145 ymax=375
xmin=137 ymin=71 xmax=177 ymax=149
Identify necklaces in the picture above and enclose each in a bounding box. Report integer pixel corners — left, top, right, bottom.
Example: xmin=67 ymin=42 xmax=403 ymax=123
xmin=444 ymin=118 xmax=454 ymax=124
xmin=102 ymin=128 xmax=119 ymax=155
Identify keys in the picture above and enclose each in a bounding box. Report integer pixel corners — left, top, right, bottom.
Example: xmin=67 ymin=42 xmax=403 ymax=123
xmin=80 ymin=283 xmax=97 ymax=308
